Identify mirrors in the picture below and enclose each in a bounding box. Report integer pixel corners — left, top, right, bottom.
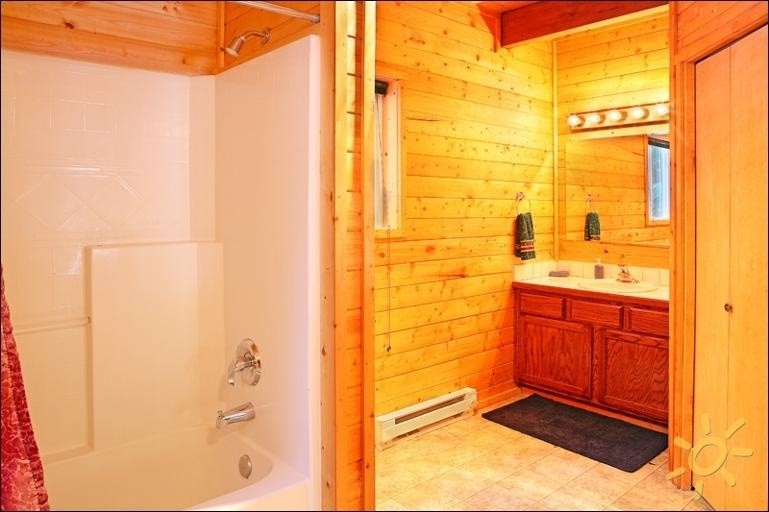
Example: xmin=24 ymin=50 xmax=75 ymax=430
xmin=565 ymin=133 xmax=671 ymax=246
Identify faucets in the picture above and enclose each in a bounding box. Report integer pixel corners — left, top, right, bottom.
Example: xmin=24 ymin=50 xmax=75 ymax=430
xmin=217 ymin=402 xmax=253 ymax=429
xmin=616 ymin=270 xmax=636 ymax=283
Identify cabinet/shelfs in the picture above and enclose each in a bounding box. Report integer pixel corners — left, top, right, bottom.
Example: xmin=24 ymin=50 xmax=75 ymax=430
xmin=514 ymin=288 xmax=668 ymax=436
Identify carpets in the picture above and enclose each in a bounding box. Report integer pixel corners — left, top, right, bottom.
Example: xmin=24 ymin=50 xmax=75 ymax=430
xmin=481 ymin=392 xmax=669 ymax=472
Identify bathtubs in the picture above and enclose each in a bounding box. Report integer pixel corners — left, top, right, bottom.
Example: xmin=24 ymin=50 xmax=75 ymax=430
xmin=40 ymin=422 xmax=310 ymax=510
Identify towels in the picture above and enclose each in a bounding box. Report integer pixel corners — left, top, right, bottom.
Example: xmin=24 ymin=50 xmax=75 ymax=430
xmin=513 ymin=212 xmax=536 ymax=261
xmin=584 ymin=211 xmax=600 ymax=240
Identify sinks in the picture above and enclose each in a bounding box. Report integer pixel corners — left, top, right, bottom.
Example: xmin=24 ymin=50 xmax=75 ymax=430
xmin=578 ymin=279 xmax=657 ymax=293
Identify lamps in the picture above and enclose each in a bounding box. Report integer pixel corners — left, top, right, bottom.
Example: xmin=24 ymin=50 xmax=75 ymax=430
xmin=566 ymin=101 xmax=670 ymax=133
